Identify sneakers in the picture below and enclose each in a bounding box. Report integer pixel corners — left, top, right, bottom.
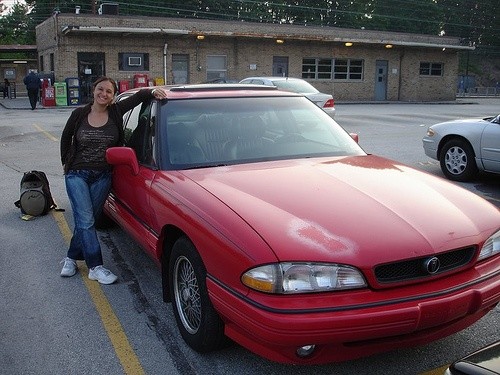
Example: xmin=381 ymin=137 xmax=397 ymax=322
xmin=60 ymin=257 xmax=78 ymax=277
xmin=87 ymin=265 xmax=118 ymax=284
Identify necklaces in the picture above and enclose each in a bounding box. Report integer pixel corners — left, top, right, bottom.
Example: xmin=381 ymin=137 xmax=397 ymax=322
xmin=92 ymin=104 xmax=107 ymax=113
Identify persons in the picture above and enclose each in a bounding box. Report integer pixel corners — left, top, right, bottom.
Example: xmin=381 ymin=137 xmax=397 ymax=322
xmin=23 ymin=70 xmax=43 ymax=110
xmin=59 ymin=77 xmax=166 ymax=284
xmin=3 ymin=78 xmax=10 ymax=99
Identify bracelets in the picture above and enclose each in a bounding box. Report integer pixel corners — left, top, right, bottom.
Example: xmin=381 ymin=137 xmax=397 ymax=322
xmin=151 ymin=89 xmax=156 ymax=96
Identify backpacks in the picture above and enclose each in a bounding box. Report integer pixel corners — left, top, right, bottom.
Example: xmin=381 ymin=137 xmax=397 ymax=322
xmin=14 ymin=170 xmax=65 ymax=217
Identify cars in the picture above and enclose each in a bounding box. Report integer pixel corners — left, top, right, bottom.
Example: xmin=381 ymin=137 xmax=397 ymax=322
xmin=97 ymin=83 xmax=500 ymax=366
xmin=421 ymin=111 xmax=500 ymax=182
xmin=238 ymin=77 xmax=336 ymax=127
